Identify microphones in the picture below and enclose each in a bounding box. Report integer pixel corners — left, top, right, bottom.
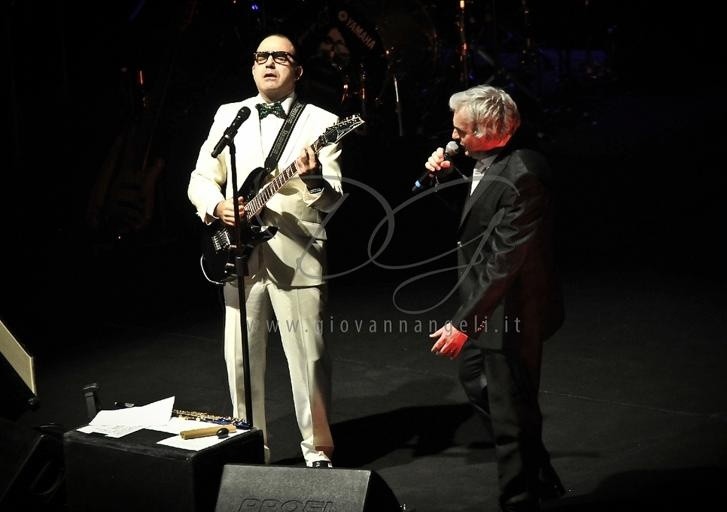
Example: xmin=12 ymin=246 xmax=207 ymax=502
xmin=210 ymin=106 xmax=251 ymax=159
xmin=411 ymin=141 xmax=460 ymax=193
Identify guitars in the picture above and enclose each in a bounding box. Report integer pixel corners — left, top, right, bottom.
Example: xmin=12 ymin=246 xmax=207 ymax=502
xmin=200 ymin=114 xmax=366 ymax=285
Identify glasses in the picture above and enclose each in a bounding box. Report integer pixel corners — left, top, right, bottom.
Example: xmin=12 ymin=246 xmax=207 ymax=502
xmin=252 ymin=51 xmax=298 ymax=65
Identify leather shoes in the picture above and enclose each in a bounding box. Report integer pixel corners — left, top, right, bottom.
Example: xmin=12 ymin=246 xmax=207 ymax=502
xmin=495 ymin=466 xmax=567 ymax=512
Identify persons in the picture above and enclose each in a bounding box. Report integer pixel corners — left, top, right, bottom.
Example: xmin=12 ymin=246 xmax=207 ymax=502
xmin=184 ymin=27 xmax=345 ymax=471
xmin=420 ymin=83 xmax=572 ymax=512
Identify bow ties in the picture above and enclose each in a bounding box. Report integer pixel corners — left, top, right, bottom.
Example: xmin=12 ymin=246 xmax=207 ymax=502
xmin=255 ymin=100 xmax=289 ymax=120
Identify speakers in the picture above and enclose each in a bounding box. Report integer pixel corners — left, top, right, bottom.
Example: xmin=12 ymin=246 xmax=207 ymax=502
xmin=214 ymin=463 xmax=403 ymax=512
xmin=0 ymin=417 xmax=63 ymax=512
